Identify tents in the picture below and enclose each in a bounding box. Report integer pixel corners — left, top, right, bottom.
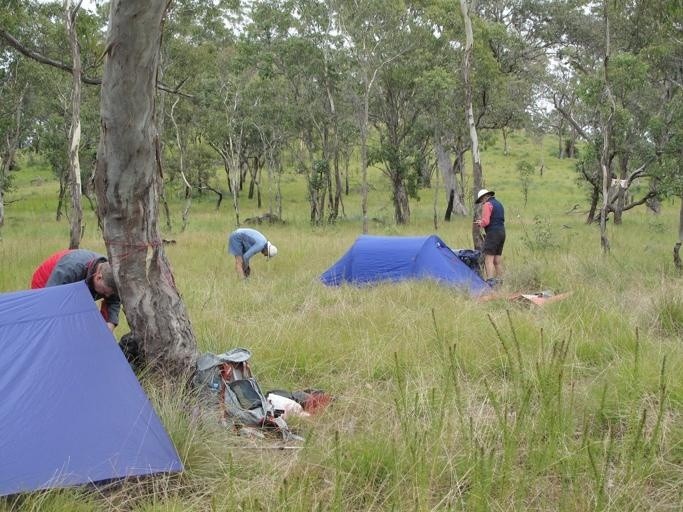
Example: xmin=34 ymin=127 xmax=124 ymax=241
xmin=317 ymin=233 xmax=497 ymax=298
xmin=1 ymin=281 xmax=184 ymax=499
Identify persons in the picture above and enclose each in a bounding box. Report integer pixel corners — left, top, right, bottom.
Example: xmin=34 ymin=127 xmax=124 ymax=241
xmin=28 ymin=248 xmax=123 ymax=334
xmin=474 ymin=189 xmax=505 ymax=286
xmin=228 ymin=228 xmax=279 ymax=278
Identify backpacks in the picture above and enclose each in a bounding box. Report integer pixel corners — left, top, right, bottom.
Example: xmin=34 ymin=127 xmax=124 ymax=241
xmin=196 ymin=348 xmax=291 ymax=443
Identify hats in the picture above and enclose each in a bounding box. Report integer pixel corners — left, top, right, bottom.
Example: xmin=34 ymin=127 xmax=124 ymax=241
xmin=267 ymin=241 xmax=277 ymax=259
xmin=475 ymin=189 xmax=495 ymax=203
